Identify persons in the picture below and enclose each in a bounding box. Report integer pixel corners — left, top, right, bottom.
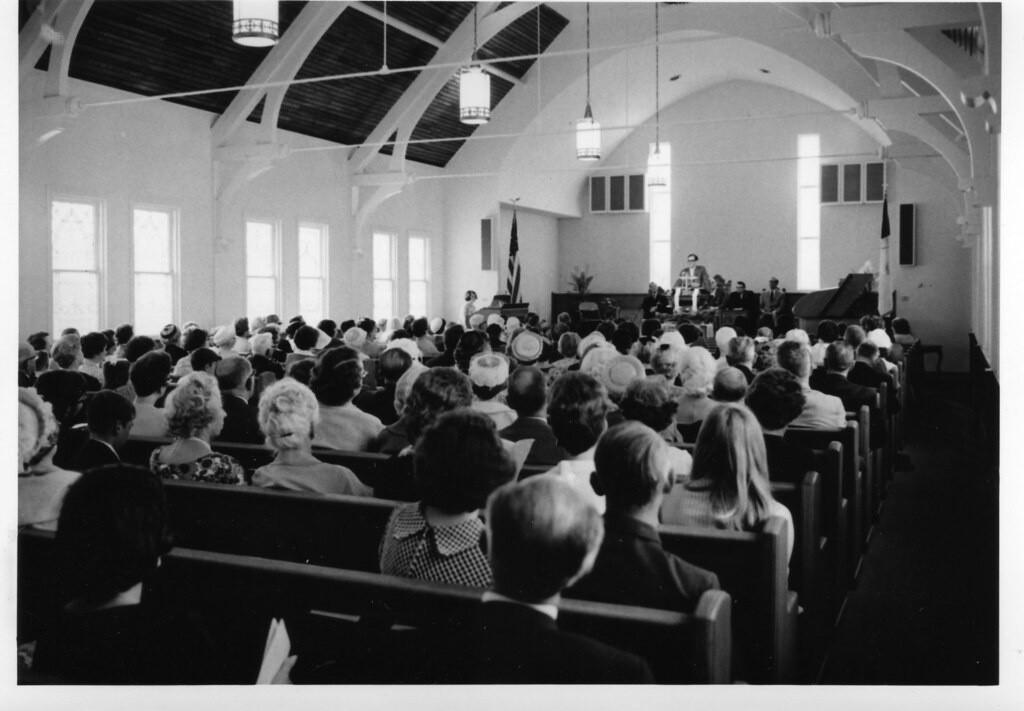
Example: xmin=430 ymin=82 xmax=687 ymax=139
xmin=674 ymin=254 xmax=711 ymax=291
xmin=16 ymin=275 xmax=916 ymax=686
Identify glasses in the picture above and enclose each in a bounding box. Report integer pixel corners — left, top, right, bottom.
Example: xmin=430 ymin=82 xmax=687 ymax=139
xmin=687 ymin=259 xmax=696 ymax=262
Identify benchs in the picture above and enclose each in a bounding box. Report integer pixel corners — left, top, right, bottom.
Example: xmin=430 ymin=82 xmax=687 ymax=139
xmin=17 ymin=338 xmax=930 ymax=685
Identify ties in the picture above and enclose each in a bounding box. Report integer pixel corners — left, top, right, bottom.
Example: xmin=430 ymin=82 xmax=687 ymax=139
xmin=770 ymin=290 xmax=773 ymax=303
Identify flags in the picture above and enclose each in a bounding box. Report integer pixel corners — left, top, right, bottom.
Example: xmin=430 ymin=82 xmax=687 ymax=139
xmin=507 ymin=211 xmax=521 ymax=302
xmin=880 ymin=193 xmax=890 ymax=237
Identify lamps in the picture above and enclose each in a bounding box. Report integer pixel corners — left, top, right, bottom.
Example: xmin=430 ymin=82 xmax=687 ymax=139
xmin=575 ymin=2 xmax=602 ymax=161
xmin=458 ymin=1 xmax=492 ymax=125
xmin=646 ymin=2 xmax=669 ymax=188
xmin=231 ymin=0 xmax=281 ymax=48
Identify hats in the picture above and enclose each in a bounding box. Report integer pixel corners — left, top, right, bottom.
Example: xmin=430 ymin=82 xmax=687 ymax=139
xmin=868 ymin=328 xmax=892 ymax=349
xmin=511 ymin=329 xmax=543 ymax=361
xmin=212 ymin=325 xmax=234 ymax=344
xmin=344 ymin=327 xmax=366 ymax=346
xmin=430 ymin=317 xmax=442 ymax=333
xmin=469 ymin=353 xmax=509 ymax=388
xmin=18 ymin=386 xmax=58 ymax=472
xmin=160 ymin=324 xmax=180 ymax=343
xmin=19 ymin=342 xmax=37 ymax=364
xmin=603 ymin=355 xmax=646 ymax=396
xmin=559 ymin=332 xmax=581 ymax=354
